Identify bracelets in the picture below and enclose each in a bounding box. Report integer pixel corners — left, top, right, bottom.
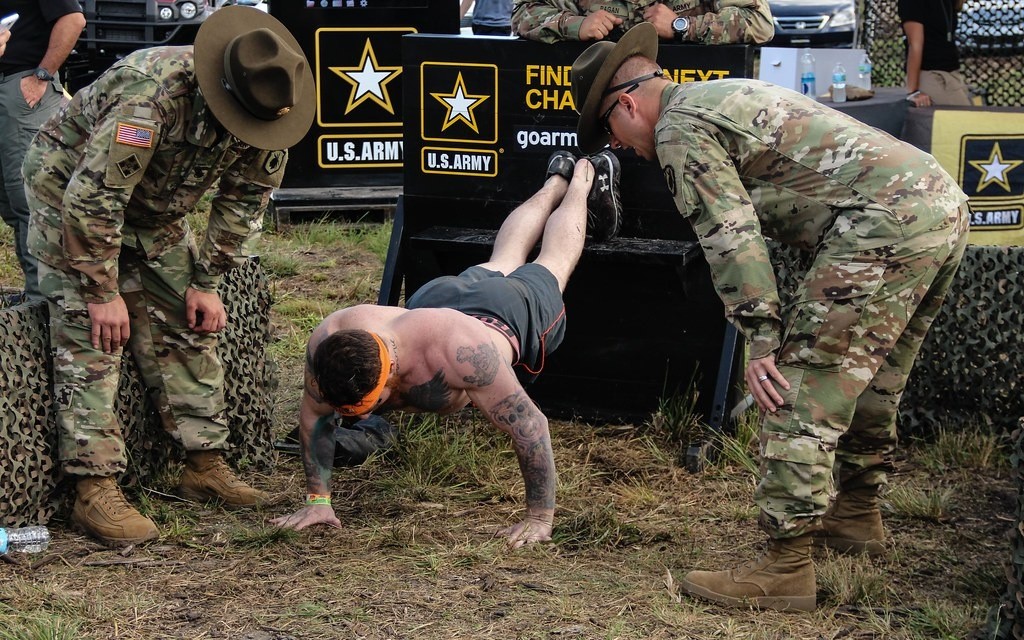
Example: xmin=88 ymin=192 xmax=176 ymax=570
xmin=906 ymin=90 xmax=920 ymax=100
xmin=306 ymin=493 xmax=331 ymax=505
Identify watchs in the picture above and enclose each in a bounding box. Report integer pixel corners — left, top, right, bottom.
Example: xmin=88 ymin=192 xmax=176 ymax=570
xmin=35 ymin=67 xmax=55 ymax=82
xmin=671 ymin=16 xmax=689 ymax=40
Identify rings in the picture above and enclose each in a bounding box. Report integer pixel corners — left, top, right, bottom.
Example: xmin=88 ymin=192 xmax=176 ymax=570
xmin=757 ymin=376 xmax=767 ymax=382
xmin=921 ymin=104 xmax=924 ymax=106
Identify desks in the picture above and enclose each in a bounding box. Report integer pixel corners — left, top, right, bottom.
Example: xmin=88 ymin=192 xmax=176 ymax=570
xmin=817 ymin=87 xmax=908 ymax=144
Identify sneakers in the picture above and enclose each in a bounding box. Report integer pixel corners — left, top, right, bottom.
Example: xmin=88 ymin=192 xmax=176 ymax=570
xmin=587 ymin=150 xmax=622 ymax=242
xmin=545 ymin=150 xmax=577 ymax=184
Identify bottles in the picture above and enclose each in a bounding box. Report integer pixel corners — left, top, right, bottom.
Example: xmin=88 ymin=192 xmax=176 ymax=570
xmin=801 ymin=48 xmax=816 ymax=100
xmin=832 ymin=62 xmax=847 ymax=102
xmin=858 ymin=53 xmax=871 ymax=90
xmin=0 ymin=526 xmax=52 ymax=553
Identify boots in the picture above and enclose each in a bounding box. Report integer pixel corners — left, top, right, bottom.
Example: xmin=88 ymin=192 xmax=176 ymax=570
xmin=70 ymin=475 xmax=158 ymax=547
xmin=179 ymin=450 xmax=269 ymax=510
xmin=818 ymin=489 xmax=884 ymax=556
xmin=683 ymin=536 xmax=816 ymax=611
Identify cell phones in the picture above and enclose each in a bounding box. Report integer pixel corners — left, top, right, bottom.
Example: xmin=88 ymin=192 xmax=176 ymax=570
xmin=0 ymin=13 xmax=19 ymax=32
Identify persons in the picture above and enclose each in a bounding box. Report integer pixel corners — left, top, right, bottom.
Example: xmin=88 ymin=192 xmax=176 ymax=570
xmin=269 ymin=151 xmax=622 ymax=551
xmin=899 ymin=0 xmax=975 ymax=108
xmin=510 ymin=0 xmax=775 ymax=44
xmin=0 ymin=0 xmax=87 ymax=300
xmin=460 ymin=0 xmax=514 ymax=35
xmin=22 ymin=4 xmax=317 ymax=546
xmin=571 ymin=23 xmax=971 ymax=613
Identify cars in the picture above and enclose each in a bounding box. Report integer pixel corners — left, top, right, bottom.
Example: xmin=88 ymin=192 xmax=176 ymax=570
xmin=957 ymin=3 xmax=1024 ymax=52
xmin=759 ymin=0 xmax=858 ymax=54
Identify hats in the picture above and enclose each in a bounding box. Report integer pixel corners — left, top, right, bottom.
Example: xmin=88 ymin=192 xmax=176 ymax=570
xmin=571 ymin=22 xmax=658 ymax=154
xmin=194 ymin=6 xmax=316 ymax=151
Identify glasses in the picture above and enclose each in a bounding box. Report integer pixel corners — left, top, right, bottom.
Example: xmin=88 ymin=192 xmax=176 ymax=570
xmin=600 ymin=83 xmax=639 ymax=148
